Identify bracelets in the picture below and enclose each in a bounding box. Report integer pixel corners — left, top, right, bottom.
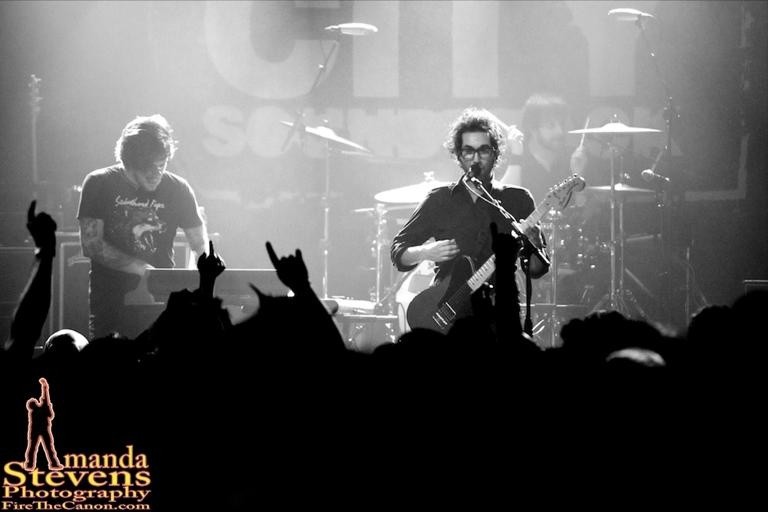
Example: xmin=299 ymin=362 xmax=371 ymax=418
xmin=508 ymin=154 xmax=524 ymax=167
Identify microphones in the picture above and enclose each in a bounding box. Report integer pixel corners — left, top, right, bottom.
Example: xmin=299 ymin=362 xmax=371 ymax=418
xmin=607 ymin=8 xmax=654 ymax=22
xmin=462 ymin=164 xmax=482 ymax=182
xmin=326 ymin=22 xmax=378 ymax=36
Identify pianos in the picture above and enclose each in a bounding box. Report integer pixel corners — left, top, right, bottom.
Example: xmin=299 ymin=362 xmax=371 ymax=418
xmin=123 ymin=270 xmax=289 ymax=343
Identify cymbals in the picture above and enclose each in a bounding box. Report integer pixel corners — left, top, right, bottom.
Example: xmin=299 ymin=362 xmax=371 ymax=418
xmin=280 ymin=122 xmax=366 ymax=151
xmin=375 ymin=183 xmax=454 ymax=203
xmin=588 ymin=183 xmax=654 ymax=193
xmin=570 ymin=122 xmax=661 ymax=134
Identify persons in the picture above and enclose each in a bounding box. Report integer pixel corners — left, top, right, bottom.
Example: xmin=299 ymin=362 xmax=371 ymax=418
xmin=500 ymin=88 xmax=596 ymax=225
xmin=390 ymin=106 xmax=551 ymax=341
xmin=2 ymin=197 xmax=767 ymax=512
xmin=75 ymin=113 xmax=211 ymax=339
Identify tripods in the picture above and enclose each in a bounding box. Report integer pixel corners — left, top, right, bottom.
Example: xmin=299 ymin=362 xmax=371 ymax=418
xmin=346 ymin=216 xmax=397 ymax=348
xmin=524 ymin=147 xmax=709 ymax=347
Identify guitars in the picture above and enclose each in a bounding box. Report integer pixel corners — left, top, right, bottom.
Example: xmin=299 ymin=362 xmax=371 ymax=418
xmin=407 ymin=175 xmax=585 ymax=334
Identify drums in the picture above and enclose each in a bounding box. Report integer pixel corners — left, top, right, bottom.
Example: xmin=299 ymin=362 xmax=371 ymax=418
xmin=531 ymin=219 xmax=581 ymax=275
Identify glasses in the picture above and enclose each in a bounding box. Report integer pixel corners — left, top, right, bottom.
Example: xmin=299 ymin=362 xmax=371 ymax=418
xmin=457 ymin=147 xmax=496 ymax=161
